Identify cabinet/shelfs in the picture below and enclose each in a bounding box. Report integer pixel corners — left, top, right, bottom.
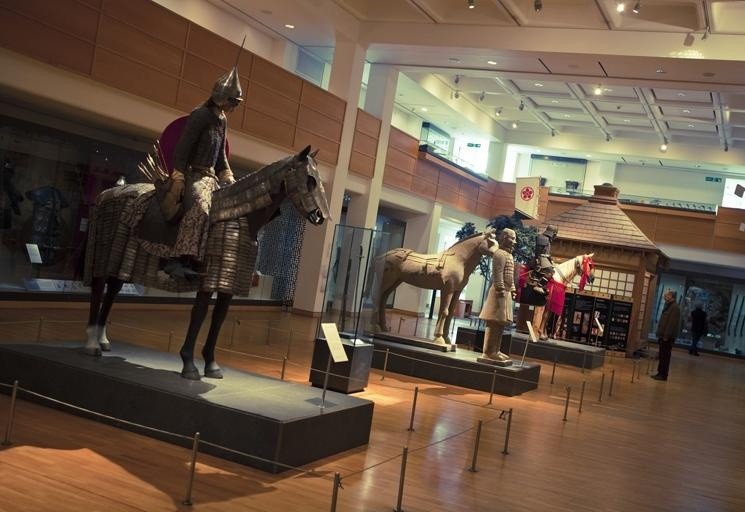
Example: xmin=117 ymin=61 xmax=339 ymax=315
xmin=518 ymin=289 xmax=634 ymax=358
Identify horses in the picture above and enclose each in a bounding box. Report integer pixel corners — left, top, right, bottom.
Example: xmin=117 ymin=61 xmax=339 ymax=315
xmin=81 ymin=142 xmax=331 ymax=381
xmin=368 ymin=227 xmax=500 ymax=346
xmin=512 ymin=251 xmax=596 ymax=342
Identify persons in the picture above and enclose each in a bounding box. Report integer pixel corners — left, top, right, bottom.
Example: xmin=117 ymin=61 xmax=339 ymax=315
xmin=478 ymin=228 xmax=516 ymax=362
xmin=688 ymin=302 xmax=709 ymax=356
xmin=153 ymin=67 xmax=243 ymax=279
xmin=651 ymin=289 xmax=682 ymax=381
xmin=533 ymin=225 xmax=558 ymax=295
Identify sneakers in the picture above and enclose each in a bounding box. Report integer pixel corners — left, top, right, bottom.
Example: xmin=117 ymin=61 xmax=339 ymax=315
xmin=651 ymin=374 xmax=667 ymax=381
xmin=688 ymin=349 xmax=701 ymax=356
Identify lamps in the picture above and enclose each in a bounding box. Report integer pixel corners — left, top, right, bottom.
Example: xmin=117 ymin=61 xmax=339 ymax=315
xmin=454 ymin=90 xmax=460 ymax=100
xmin=479 ymin=91 xmax=486 ymax=101
xmin=702 ymin=26 xmax=711 ymax=41
xmin=466 ymin=0 xmax=476 ymax=9
xmin=517 ymin=100 xmax=526 ymax=111
xmin=533 ymin=0 xmax=542 ymax=12
xmin=495 ymin=109 xmax=503 ymax=116
xmin=454 ymin=74 xmax=460 ymax=84
xmin=632 ymin=0 xmax=640 ymax=15
